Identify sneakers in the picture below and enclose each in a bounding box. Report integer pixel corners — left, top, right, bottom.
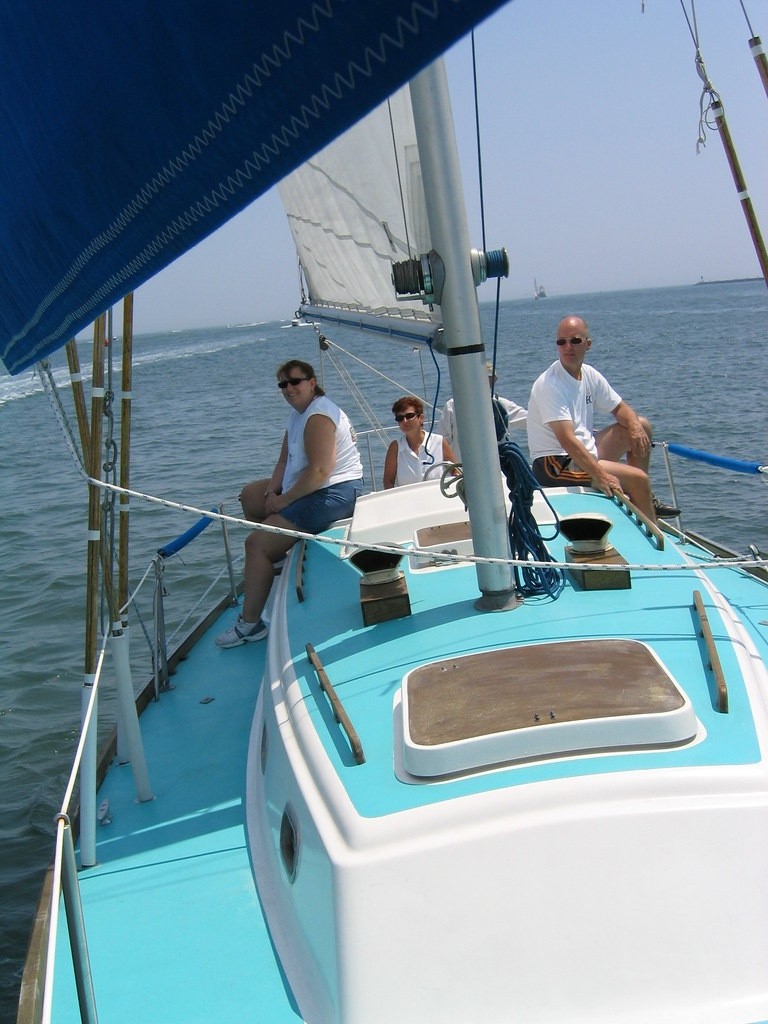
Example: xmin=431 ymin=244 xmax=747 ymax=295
xmin=214 ymin=614 xmax=268 ymax=648
xmin=272 ymin=554 xmax=288 ymax=574
xmin=652 ymin=490 xmax=682 ymax=519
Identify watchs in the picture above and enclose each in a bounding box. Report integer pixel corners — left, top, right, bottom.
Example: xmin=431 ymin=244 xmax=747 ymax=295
xmin=264 ymin=489 xmax=277 ymax=498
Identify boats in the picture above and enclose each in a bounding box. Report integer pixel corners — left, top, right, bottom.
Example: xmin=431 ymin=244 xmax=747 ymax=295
xmin=291 ymin=318 xmax=301 ymax=326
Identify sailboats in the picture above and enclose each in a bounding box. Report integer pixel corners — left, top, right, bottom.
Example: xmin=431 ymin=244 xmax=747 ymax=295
xmin=534 ymin=278 xmax=547 ymax=300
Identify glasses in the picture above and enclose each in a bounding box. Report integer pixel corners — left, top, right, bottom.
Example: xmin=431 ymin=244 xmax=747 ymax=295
xmin=278 ymin=378 xmax=310 ymax=388
xmin=556 ymin=338 xmax=586 ymax=345
xmin=395 ymin=413 xmax=420 ymax=421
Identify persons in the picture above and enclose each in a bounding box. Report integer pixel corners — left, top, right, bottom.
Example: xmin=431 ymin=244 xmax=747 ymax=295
xmin=437 ymin=359 xmax=528 ymax=480
xmin=526 ymin=317 xmax=681 ymax=527
xmin=215 ymin=360 xmax=364 ymax=649
xmin=383 ymin=397 xmax=460 ymax=490
